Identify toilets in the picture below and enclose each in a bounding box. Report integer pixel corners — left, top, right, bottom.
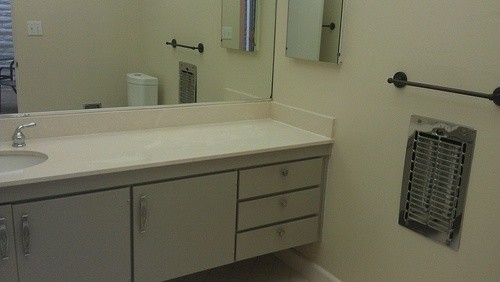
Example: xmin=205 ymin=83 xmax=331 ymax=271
xmin=126 ymin=72 xmax=159 ymax=107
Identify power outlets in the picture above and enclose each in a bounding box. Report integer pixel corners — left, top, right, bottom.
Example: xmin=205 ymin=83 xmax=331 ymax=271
xmin=25 ymin=20 xmax=43 ymax=36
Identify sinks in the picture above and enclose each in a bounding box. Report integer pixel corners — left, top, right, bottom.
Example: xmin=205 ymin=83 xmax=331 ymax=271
xmin=0 ymin=149 xmax=49 ymax=173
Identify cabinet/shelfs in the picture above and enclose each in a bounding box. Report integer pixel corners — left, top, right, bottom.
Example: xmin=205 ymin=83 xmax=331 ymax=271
xmin=0 ymin=144 xmax=333 ymax=282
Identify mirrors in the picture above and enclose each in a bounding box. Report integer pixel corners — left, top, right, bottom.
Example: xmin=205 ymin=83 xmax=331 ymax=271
xmin=0 ymin=0 xmax=278 ymax=115
